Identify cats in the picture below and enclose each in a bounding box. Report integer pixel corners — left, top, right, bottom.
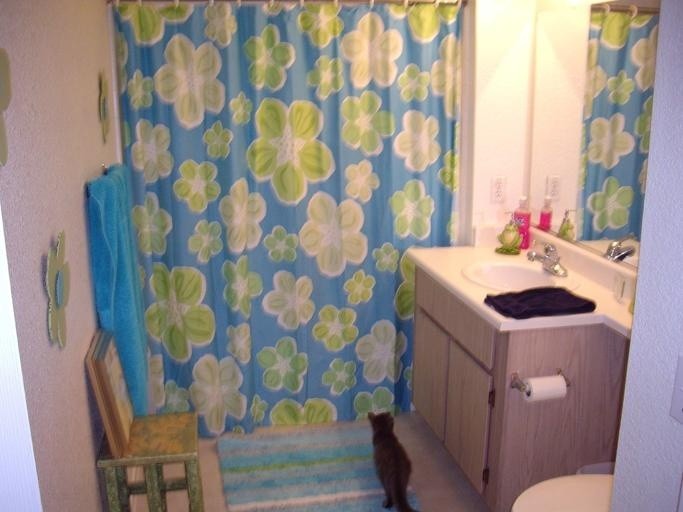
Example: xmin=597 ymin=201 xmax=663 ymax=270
xmin=367 ymin=411 xmax=420 ymax=512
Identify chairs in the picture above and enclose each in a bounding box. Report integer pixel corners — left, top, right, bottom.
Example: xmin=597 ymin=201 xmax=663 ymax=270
xmin=84 ymin=328 xmax=204 ymax=511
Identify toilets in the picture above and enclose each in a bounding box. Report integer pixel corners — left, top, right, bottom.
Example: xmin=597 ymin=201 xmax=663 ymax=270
xmin=511 ymin=473 xmax=614 ymax=512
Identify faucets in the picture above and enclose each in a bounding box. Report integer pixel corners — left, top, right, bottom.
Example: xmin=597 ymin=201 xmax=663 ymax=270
xmin=603 ymin=237 xmax=635 ymax=262
xmin=526 ymin=242 xmax=567 ymax=277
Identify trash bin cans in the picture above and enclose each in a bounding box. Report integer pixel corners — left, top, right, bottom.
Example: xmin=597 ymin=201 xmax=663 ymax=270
xmin=576 ymin=462 xmax=614 ymax=475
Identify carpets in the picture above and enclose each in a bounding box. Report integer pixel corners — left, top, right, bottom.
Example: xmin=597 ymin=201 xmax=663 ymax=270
xmin=213 ymin=426 xmax=423 ymax=511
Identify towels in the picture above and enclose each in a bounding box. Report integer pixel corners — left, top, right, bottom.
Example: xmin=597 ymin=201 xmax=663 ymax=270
xmin=483 ymin=286 xmax=596 ymax=319
xmin=83 ymin=162 xmax=149 ymax=417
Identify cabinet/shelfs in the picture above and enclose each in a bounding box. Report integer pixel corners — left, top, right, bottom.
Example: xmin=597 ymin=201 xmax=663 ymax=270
xmin=412 ymin=265 xmax=614 ymax=512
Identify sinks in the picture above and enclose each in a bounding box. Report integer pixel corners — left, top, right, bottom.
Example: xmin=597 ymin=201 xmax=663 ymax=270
xmin=622 ymin=256 xmax=639 ymax=266
xmin=462 ymin=256 xmax=558 ymax=295
